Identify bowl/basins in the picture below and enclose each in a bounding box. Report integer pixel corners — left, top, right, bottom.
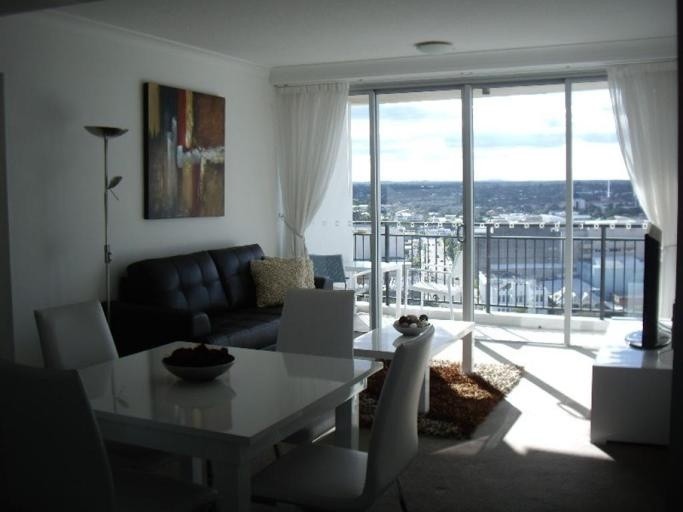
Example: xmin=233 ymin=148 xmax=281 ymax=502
xmin=160 ymin=353 xmax=236 ymax=384
xmin=392 ymin=322 xmax=430 ymax=336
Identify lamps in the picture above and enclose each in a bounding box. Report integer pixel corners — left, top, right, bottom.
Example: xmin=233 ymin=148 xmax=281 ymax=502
xmin=84 ymin=122 xmax=130 ymax=320
xmin=417 ymin=43 xmax=454 ymax=56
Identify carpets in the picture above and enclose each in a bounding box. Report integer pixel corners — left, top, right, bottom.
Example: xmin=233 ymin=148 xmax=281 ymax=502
xmin=360 ymin=359 xmax=523 ymax=441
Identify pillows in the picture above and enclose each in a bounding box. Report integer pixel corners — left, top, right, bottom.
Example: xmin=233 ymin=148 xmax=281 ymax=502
xmin=248 ymin=256 xmax=313 ymax=307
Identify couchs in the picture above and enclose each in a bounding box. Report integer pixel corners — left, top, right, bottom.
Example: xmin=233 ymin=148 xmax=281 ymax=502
xmin=115 ymin=245 xmax=332 ymax=352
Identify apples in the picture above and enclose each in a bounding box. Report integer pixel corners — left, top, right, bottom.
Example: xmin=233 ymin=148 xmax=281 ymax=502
xmin=399 ymin=313 xmax=427 ymax=329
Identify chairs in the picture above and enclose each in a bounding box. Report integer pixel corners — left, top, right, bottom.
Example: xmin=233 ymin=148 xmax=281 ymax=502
xmin=412 ymin=251 xmax=464 ymax=320
xmin=34 ymin=299 xmax=214 ymax=487
xmin=254 ymin=288 xmax=356 ymax=459
xmin=1 ymin=362 xmax=115 ymax=508
xmin=251 ymin=321 xmax=435 ymax=508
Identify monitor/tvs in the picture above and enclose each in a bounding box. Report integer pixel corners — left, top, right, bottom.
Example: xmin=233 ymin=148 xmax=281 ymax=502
xmin=625 ymin=223 xmax=671 ymax=348
